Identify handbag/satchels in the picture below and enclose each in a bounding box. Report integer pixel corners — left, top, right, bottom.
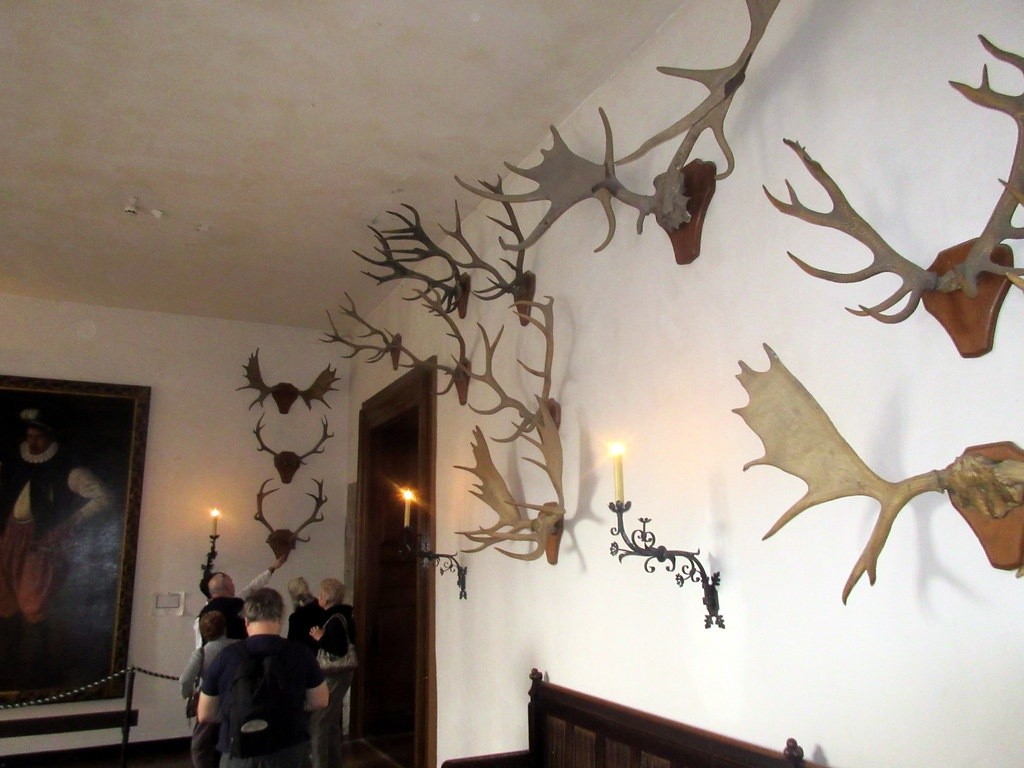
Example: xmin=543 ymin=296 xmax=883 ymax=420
xmin=315 ymin=614 xmax=358 ymax=671
xmin=185 ymin=647 xmax=204 ymax=718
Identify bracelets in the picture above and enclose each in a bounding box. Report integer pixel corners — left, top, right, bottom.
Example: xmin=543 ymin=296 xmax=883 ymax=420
xmin=268 ymin=567 xmax=275 ymax=573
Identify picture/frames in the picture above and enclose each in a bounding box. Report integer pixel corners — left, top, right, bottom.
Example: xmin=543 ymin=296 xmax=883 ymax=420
xmin=0 ymin=373 xmax=155 ymax=710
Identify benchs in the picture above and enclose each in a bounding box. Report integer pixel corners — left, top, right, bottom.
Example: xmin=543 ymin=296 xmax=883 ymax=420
xmin=441 ymin=668 xmax=829 ymax=768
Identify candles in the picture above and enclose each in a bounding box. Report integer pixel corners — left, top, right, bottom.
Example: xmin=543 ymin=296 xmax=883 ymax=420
xmin=609 ymin=441 xmax=625 ymax=503
xmin=403 ymin=490 xmax=413 ymax=526
xmin=210 ymin=508 xmax=220 ymax=536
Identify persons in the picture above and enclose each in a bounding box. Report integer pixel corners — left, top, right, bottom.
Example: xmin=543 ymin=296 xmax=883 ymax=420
xmin=179 ymin=555 xmax=356 ymax=768
xmin=198 ymin=587 xmax=329 ymax=768
